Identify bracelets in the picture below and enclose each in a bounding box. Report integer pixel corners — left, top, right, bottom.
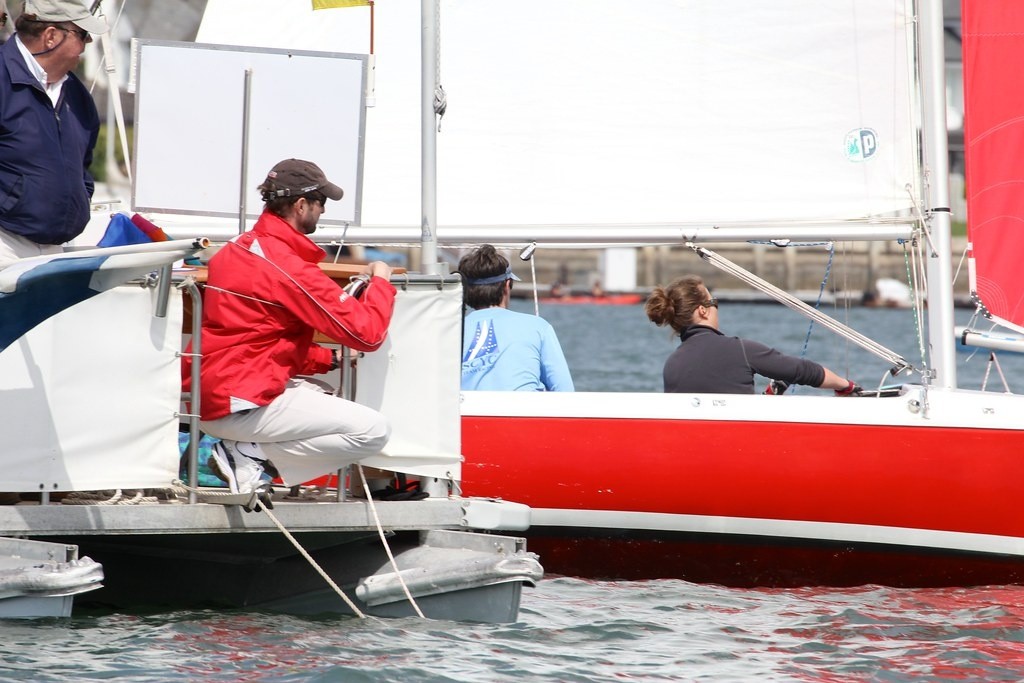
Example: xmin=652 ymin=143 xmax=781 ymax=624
xmin=834 ymin=381 xmax=853 ymax=395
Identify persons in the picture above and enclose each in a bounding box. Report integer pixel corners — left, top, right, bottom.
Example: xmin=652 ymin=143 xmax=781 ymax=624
xmin=184 ymin=158 xmax=396 ymax=493
xmin=552 ymin=281 xmax=566 ymax=298
xmin=459 ymin=245 xmax=575 ymax=391
xmin=591 ymin=279 xmax=607 ymax=297
xmin=0 ymin=0 xmax=109 ymax=265
xmin=646 ymin=274 xmax=857 ymax=397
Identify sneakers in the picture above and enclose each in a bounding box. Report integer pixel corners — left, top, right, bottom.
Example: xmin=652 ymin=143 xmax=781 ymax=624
xmin=212 ymin=439 xmax=265 ymax=494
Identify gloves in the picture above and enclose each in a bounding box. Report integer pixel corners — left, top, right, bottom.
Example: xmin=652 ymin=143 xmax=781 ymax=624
xmin=763 ymin=378 xmax=789 ymax=395
xmin=835 ymin=381 xmax=863 ymax=397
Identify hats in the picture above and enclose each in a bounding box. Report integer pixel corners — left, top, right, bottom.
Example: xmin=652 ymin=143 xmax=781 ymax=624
xmin=263 ymin=158 xmax=343 ymax=201
xmin=24 ymin=0 xmax=110 ymax=35
xmin=468 ymin=266 xmax=522 ymax=285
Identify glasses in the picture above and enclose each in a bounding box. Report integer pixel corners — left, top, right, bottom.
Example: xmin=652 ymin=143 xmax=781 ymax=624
xmin=305 ymin=196 xmax=327 ymax=206
xmin=704 ymin=297 xmax=718 ymax=308
xmin=60 ymin=27 xmax=88 ymax=42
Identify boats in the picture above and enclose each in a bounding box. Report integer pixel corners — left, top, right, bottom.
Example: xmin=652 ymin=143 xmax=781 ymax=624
xmin=531 ymin=293 xmax=645 ymax=305
xmin=0 ymin=235 xmax=540 ymax=627
xmin=862 ymin=276 xmax=926 ymax=310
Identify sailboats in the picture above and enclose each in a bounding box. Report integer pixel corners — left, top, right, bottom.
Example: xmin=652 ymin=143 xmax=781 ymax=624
xmin=170 ymin=2 xmax=1024 ymax=555
